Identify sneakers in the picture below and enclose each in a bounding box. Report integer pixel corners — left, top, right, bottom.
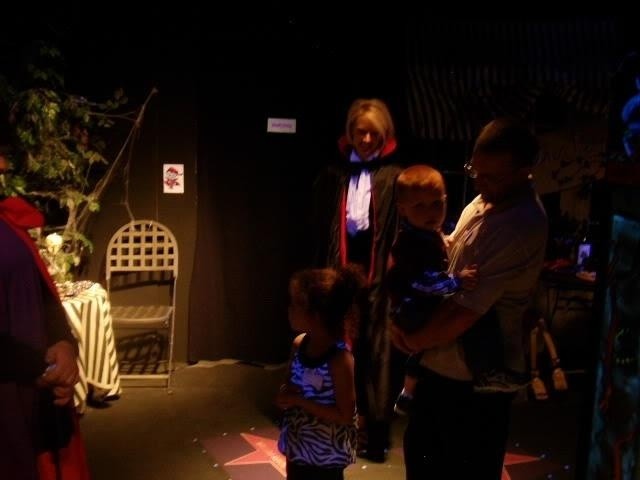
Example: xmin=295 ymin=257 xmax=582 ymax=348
xmin=393 ymin=392 xmax=413 ymax=414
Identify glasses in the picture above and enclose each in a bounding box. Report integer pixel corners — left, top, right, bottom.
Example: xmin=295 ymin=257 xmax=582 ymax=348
xmin=462 ymin=163 xmax=480 ymax=177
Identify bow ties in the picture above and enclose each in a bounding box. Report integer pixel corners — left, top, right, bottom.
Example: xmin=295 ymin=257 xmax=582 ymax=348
xmin=348 ymin=158 xmax=375 ymax=177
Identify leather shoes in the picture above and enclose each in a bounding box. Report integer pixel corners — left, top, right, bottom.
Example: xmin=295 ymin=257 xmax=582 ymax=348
xmin=356 ymin=443 xmax=388 ymax=462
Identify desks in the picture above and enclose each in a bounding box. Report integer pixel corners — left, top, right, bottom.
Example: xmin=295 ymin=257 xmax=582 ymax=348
xmin=54 ymin=281 xmax=107 ymax=417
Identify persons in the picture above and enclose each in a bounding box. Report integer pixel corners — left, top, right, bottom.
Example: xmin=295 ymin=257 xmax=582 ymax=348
xmin=1 ymin=156 xmax=80 ymax=479
xmin=402 ymin=118 xmax=548 ymax=480
xmin=322 ymin=97 xmax=405 ymax=463
xmin=384 ymin=165 xmax=537 ymax=415
xmin=266 ymin=263 xmax=372 ymax=480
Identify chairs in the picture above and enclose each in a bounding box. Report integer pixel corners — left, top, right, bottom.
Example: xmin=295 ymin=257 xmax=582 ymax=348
xmin=104 ymin=219 xmax=180 ymax=394
